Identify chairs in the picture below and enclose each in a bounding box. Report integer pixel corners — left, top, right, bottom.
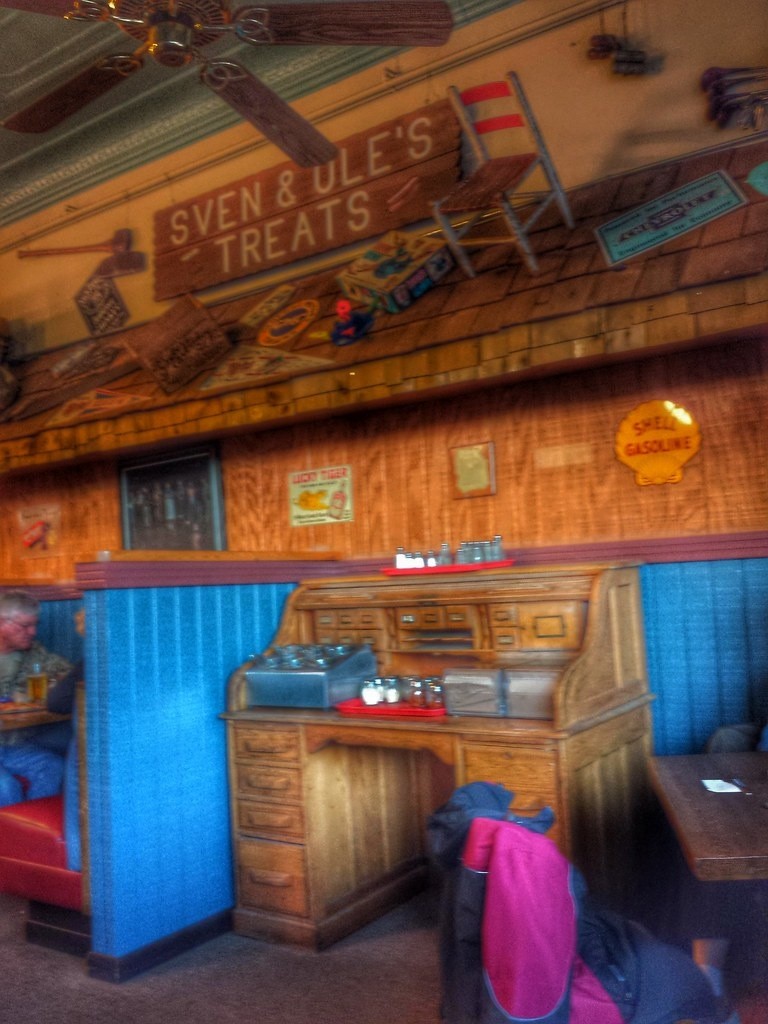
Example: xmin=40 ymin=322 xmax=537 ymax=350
xmin=428 ymin=70 xmax=575 ymax=276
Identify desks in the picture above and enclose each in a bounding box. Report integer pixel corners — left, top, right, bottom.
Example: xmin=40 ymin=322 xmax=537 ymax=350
xmin=645 ymin=754 xmax=767 ymax=1002
xmin=217 ymin=558 xmax=653 ymax=952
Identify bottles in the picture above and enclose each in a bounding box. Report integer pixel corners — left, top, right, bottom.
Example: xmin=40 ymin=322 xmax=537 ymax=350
xmin=326 ymin=479 xmax=348 ymax=520
xmin=258 ymin=644 xmax=354 ymax=670
xmin=393 ymin=533 xmax=503 ymax=569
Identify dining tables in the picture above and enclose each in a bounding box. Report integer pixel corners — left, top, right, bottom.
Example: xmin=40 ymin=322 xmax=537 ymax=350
xmin=0 ymin=699 xmax=72 ymax=732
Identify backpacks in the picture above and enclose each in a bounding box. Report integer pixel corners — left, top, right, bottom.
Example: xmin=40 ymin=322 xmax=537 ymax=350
xmin=428 ymin=802 xmax=738 ymax=1024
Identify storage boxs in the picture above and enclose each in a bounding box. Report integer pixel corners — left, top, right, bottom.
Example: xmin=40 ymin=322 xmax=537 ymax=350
xmin=337 ymin=227 xmax=457 ymax=316
xmin=124 ymin=292 xmax=232 ymax=396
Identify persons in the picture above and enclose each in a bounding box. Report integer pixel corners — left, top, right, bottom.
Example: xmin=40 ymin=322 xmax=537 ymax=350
xmin=0 ymin=589 xmax=85 ymax=807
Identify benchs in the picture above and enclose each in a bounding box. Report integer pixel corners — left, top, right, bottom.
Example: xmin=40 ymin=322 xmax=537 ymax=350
xmin=0 ymin=681 xmax=91 ymax=914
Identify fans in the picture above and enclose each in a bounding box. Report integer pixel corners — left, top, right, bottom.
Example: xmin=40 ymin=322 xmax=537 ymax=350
xmin=0 ymin=0 xmax=454 ymax=168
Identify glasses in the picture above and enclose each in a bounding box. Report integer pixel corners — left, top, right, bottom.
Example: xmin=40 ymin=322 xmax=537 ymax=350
xmin=10 ymin=619 xmax=40 ymax=628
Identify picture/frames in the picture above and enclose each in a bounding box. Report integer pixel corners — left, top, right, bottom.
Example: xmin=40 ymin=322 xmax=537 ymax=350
xmin=116 ymin=439 xmax=226 ymax=550
